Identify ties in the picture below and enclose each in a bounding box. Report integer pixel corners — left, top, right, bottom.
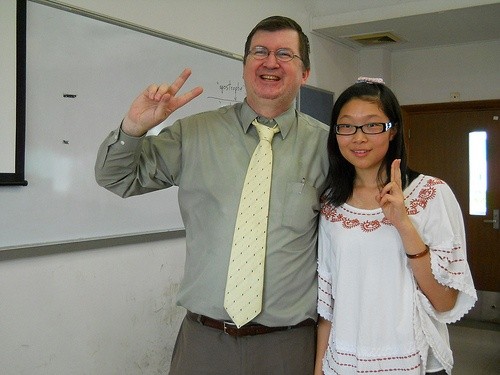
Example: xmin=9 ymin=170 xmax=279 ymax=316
xmin=223 ymin=118 xmax=281 ymax=329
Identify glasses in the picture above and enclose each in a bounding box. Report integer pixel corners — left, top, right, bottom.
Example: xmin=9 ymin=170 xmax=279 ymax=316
xmin=335 ymin=122 xmax=392 ymax=136
xmin=246 ymin=46 xmax=303 ymax=62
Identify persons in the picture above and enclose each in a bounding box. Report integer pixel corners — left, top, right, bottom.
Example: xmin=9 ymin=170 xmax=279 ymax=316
xmin=95 ymin=15 xmax=331 ymax=375
xmin=314 ymin=77 xmax=477 ymax=375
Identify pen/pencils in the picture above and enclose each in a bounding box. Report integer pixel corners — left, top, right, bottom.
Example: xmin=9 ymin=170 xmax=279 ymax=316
xmin=300 ymin=178 xmax=305 ymax=195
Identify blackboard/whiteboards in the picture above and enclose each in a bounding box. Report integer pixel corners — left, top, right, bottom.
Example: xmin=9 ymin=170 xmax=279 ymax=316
xmin=0 ymin=0 xmax=300 ymax=251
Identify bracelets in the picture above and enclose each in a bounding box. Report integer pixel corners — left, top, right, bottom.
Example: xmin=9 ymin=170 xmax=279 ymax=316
xmin=405 ymin=244 xmax=430 ymax=260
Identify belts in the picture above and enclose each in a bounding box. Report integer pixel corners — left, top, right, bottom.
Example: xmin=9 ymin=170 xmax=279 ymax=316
xmin=201 ymin=317 xmax=313 ymax=337
